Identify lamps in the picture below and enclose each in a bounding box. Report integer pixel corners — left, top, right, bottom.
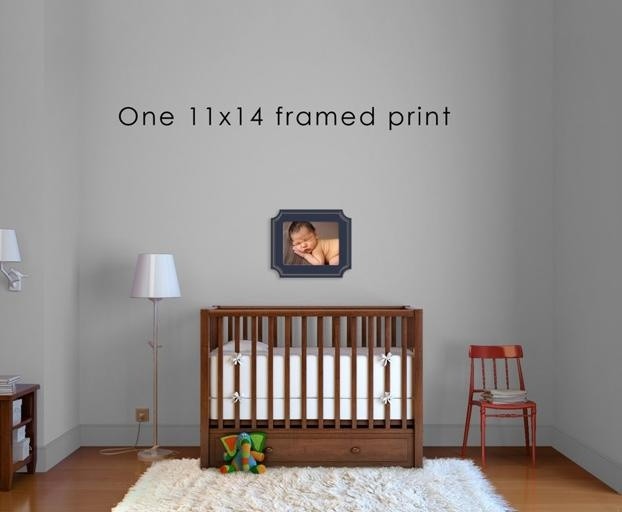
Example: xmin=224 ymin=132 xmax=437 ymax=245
xmin=132 ymin=253 xmax=181 ymax=461
xmin=2 ymin=229 xmax=31 ymax=292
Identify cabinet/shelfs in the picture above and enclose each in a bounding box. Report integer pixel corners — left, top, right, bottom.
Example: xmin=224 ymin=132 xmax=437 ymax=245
xmin=0 ymin=384 xmax=41 ymax=490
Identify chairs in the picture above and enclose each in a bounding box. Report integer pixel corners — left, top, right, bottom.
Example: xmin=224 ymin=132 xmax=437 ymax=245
xmin=460 ymin=346 xmax=538 ymax=466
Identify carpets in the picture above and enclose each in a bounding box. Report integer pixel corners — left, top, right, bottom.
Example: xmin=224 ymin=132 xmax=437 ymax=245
xmin=111 ymin=457 xmax=513 ymax=512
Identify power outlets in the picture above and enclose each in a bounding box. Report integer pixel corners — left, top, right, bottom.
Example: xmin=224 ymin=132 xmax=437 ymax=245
xmin=135 ymin=409 xmax=149 ymax=422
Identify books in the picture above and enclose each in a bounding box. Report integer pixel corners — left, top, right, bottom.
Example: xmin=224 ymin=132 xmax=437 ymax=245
xmin=0 ymin=375 xmax=24 ymax=395
xmin=480 ymin=390 xmax=529 ymax=404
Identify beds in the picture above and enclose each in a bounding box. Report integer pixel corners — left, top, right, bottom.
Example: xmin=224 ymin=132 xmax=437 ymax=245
xmin=201 ymin=306 xmax=422 ymax=470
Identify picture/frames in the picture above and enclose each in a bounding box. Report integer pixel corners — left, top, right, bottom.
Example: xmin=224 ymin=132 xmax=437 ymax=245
xmin=272 ymin=210 xmax=351 ymax=277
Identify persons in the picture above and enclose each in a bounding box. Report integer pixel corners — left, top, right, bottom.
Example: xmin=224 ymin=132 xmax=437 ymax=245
xmin=220 ymin=432 xmax=267 ymax=474
xmin=289 ymin=221 xmax=339 ymax=266
